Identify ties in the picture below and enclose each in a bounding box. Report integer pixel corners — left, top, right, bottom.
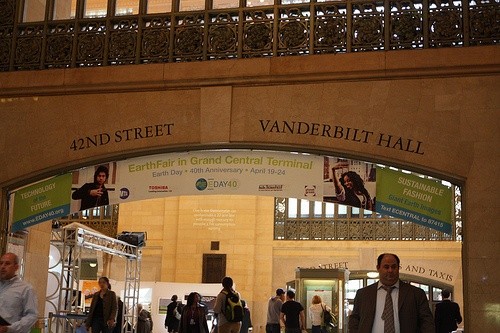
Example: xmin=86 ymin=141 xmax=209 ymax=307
xmin=382 ymin=285 xmax=396 ymax=333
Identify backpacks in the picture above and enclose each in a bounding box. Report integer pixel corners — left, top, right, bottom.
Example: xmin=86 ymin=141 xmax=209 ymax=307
xmin=221 ymin=290 xmax=244 ymax=322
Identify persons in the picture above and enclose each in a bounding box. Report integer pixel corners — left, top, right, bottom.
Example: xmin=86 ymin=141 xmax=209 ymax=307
xmin=348 ymin=253 xmax=433 ymax=333
xmin=72 ymin=165 xmax=109 ymax=211
xmin=0 ymin=253 xmax=39 ymax=333
xmin=85 ymin=276 xmax=330 ymax=333
xmin=435 ymin=288 xmax=462 ymax=333
xmin=333 ymin=162 xmax=372 ymax=210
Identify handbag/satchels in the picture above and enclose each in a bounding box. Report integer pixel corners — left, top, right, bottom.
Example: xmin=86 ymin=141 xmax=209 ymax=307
xmin=321 ymin=303 xmax=337 ymax=328
xmin=173 ymin=301 xmax=183 ymax=320
